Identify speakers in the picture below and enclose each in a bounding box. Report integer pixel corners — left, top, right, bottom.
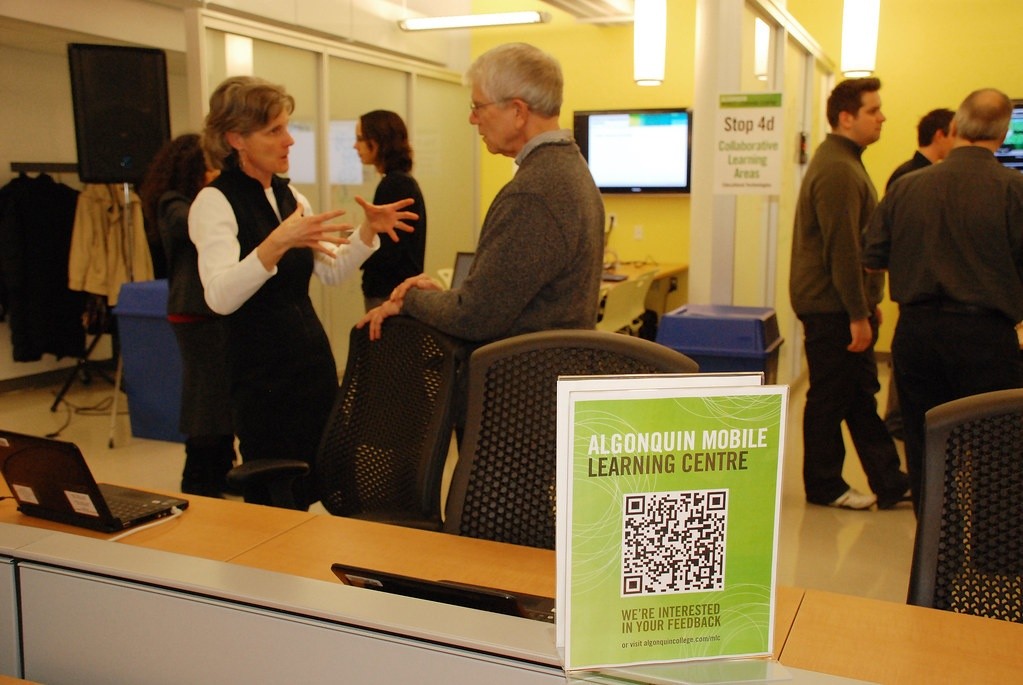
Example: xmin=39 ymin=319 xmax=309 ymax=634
xmin=69 ymin=43 xmax=172 ymax=185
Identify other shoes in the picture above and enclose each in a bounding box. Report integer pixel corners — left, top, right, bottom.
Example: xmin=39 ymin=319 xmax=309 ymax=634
xmin=830 ymin=487 xmax=878 ymax=509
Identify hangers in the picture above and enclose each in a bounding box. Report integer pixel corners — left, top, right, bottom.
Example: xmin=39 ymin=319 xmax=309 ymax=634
xmin=16 ymin=164 xmax=45 ymax=180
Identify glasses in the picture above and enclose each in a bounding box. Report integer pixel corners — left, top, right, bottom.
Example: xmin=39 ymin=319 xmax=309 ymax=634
xmin=471 ymin=97 xmax=531 ymax=117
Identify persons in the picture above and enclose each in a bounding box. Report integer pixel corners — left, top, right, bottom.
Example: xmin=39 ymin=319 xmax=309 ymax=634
xmin=356 ymin=40 xmax=605 ymax=458
xmin=885 ymin=106 xmax=958 ymax=441
xmin=353 ymin=108 xmax=427 ymax=314
xmin=860 ymin=88 xmax=1023 ymax=575
xmin=138 ymin=133 xmax=238 ymax=497
xmin=188 ymin=75 xmax=419 ymax=512
xmin=789 ymin=76 xmax=910 ymax=511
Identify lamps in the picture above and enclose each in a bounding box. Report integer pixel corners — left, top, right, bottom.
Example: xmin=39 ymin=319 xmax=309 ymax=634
xmin=399 ymin=11 xmax=550 ymax=32
xmin=840 ymin=0 xmax=880 ymax=77
xmin=633 ymin=0 xmax=667 ymax=86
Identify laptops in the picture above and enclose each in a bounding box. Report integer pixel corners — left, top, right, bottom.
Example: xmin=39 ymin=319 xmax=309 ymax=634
xmin=450 ymin=252 xmax=475 ymax=290
xmin=331 ymin=563 xmax=555 ymax=624
xmin=0 ymin=429 xmax=189 ymax=534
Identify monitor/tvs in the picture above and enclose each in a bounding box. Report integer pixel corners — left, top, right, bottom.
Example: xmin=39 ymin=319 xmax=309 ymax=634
xmin=573 ymin=111 xmax=692 ymax=194
xmin=993 ymin=98 xmax=1023 ymax=174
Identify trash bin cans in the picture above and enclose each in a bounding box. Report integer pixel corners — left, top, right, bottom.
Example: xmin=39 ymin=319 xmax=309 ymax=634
xmin=110 ymin=278 xmax=189 ymax=442
xmin=654 ymin=303 xmax=785 ymax=385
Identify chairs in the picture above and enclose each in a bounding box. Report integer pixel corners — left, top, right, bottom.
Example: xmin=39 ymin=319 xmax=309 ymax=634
xmin=906 ymin=387 xmax=1023 ymax=625
xmin=229 ymin=314 xmax=455 ymax=541
xmin=443 ymin=329 xmax=700 ymax=551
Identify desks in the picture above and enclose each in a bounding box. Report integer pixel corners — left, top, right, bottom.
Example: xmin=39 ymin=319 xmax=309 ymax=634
xmin=0 ymin=483 xmax=1023 ymax=685
